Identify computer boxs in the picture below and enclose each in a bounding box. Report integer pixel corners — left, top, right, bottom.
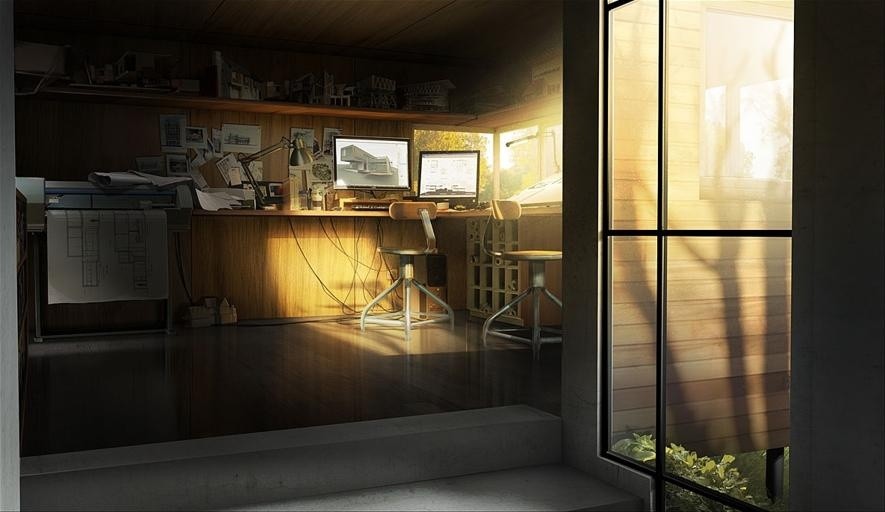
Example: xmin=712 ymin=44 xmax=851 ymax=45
xmin=425 ymin=254 xmax=448 ymax=316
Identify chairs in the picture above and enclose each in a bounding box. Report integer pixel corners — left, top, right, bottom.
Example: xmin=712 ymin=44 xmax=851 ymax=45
xmin=358 ymin=198 xmax=457 ymax=336
xmin=478 ymin=198 xmax=563 ymax=363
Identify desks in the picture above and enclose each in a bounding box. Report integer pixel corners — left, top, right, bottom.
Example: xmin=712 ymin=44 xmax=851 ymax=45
xmin=181 ymin=194 xmax=565 ymax=219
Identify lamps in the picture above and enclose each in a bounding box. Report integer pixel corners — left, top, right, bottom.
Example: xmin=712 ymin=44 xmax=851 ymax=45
xmin=238 ymin=134 xmax=315 ymax=211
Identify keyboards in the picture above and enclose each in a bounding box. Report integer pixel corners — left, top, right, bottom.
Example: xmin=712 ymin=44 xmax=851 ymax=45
xmin=350 ymin=204 xmax=394 ymax=211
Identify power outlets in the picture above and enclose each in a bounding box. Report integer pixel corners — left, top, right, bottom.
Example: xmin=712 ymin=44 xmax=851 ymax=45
xmin=387 ymin=268 xmax=398 ymax=280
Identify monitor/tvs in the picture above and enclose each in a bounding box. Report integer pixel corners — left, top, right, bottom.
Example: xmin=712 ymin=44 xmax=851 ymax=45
xmin=332 ymin=134 xmax=413 ymax=194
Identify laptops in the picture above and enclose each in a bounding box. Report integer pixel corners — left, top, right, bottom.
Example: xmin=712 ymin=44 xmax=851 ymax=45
xmin=416 ymin=150 xmax=480 ymax=210
